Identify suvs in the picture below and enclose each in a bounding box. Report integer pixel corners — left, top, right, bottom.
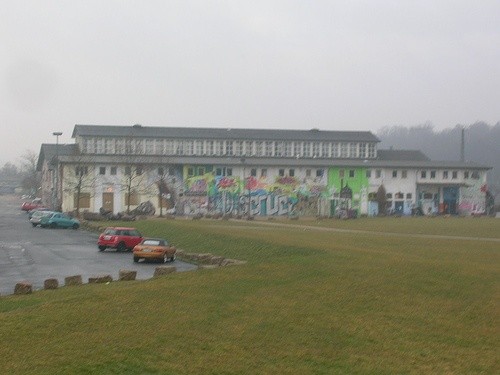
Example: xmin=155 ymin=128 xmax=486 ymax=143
xmin=96 ymin=226 xmax=144 ymax=253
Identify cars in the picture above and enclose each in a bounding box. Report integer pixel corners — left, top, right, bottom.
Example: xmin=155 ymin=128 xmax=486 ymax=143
xmin=132 ymin=238 xmax=177 ymax=263
xmin=20 ymin=196 xmax=51 ymax=227
xmin=40 ymin=212 xmax=80 ymax=230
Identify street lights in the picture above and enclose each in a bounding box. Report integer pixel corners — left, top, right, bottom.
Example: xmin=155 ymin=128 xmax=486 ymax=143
xmin=52 ymin=130 xmax=63 ymax=211
